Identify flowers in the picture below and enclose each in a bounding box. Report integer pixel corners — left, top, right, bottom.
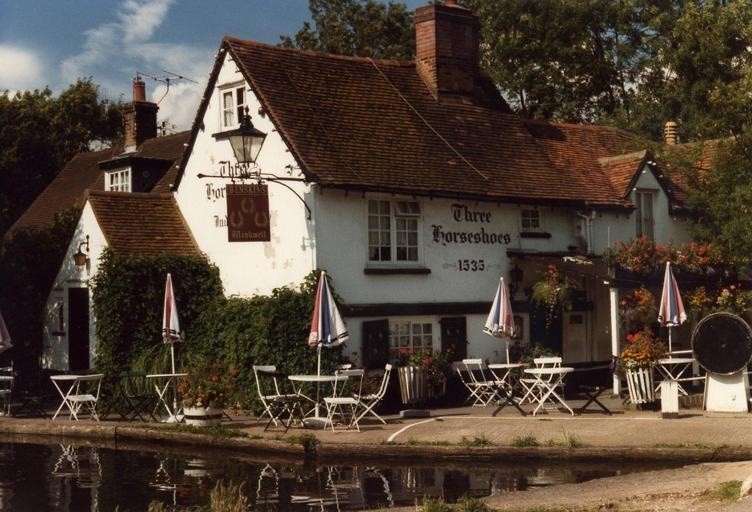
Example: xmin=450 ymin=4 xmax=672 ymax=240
xmin=177 ymin=363 xmax=240 ymax=410
xmin=528 ymin=264 xmax=582 ymax=328
xmin=617 ymin=286 xmax=752 ymax=331
xmin=397 ymin=344 xmax=437 ymax=369
xmin=610 ymin=233 xmax=730 ymax=276
xmin=619 ymin=324 xmax=671 ymax=371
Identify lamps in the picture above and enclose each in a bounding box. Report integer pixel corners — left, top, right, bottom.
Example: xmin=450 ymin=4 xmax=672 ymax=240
xmin=223 ymin=105 xmax=268 ymax=178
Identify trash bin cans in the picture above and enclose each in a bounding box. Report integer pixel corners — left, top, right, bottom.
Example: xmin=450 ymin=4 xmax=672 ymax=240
xmin=398 ymin=366 xmax=430 ymax=418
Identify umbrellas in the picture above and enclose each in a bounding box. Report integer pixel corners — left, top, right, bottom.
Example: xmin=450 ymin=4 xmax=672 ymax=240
xmin=161 ymin=268 xmax=186 ymax=412
xmin=0 ymin=306 xmax=14 ymax=352
xmin=659 ymin=260 xmax=686 ymax=381
xmin=483 ymin=277 xmax=517 ymax=400
xmin=306 ymin=272 xmax=348 ymax=416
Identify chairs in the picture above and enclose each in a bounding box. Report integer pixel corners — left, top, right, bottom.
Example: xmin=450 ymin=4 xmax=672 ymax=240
xmin=51 ymin=370 xmax=196 ymax=426
xmin=457 ymin=355 xmax=576 ymax=417
xmin=0 ymin=360 xmax=17 ymax=420
xmin=250 ymin=360 xmax=397 ymax=434
